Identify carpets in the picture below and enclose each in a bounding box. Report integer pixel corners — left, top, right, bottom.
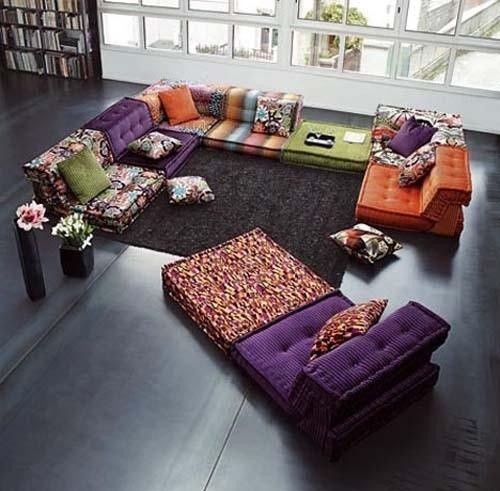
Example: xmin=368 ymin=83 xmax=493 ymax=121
xmin=107 ymin=144 xmax=366 ymax=291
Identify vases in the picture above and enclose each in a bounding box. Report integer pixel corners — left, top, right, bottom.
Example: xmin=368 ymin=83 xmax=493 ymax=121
xmin=13 ymin=228 xmax=47 ymax=303
xmin=57 ymin=241 xmax=96 ymax=280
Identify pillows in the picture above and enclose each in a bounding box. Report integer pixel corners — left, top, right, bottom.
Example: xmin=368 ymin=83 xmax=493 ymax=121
xmin=157 ymin=84 xmax=201 ymax=126
xmin=250 ymin=93 xmax=300 ymax=138
xmin=396 ymin=141 xmax=439 ymax=188
xmin=386 ymin=115 xmax=440 ymax=157
xmin=164 ymin=175 xmax=216 ymax=206
xmin=304 ymin=297 xmax=390 ymax=359
xmin=327 ymin=220 xmax=405 ymax=266
xmin=56 ymin=145 xmax=114 ymax=208
xmin=127 ymin=130 xmax=184 ymax=162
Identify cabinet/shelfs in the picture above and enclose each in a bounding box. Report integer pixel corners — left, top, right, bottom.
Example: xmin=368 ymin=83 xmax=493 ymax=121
xmin=1 ymin=0 xmax=103 ymax=82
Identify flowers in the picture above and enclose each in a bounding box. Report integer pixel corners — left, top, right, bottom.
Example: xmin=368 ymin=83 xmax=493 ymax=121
xmin=15 ymin=196 xmax=50 ymax=233
xmin=52 ymin=211 xmax=97 ymax=252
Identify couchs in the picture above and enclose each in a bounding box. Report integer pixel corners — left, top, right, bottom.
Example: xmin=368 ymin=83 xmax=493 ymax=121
xmin=21 ymin=124 xmax=170 ymax=234
xmin=367 ymin=103 xmax=467 ymax=167
xmin=203 ymin=83 xmax=304 ymax=161
xmin=158 ymin=222 xmax=341 ymax=355
xmin=355 ymin=144 xmax=475 ymax=243
xmin=232 ymin=291 xmax=452 ymax=461
xmin=78 ymin=95 xmax=201 ymax=181
xmin=279 ymin=116 xmax=375 ymax=174
xmin=122 ymin=77 xmax=232 ymax=146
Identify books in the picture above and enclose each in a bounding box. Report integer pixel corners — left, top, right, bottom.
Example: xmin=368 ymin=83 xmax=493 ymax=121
xmin=0 ymin=0 xmax=89 ymax=82
